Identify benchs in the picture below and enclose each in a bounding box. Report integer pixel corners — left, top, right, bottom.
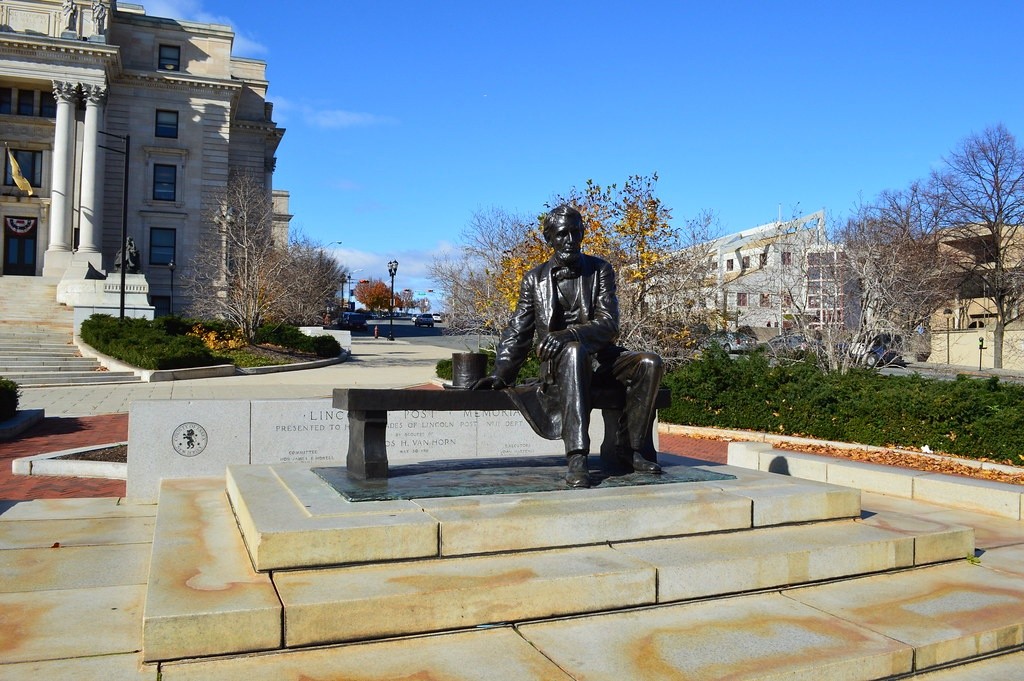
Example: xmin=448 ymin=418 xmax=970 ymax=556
xmin=332 ymin=384 xmax=671 ymax=478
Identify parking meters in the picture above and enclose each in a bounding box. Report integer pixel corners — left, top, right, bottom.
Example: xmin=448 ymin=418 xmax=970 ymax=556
xmin=978 ymin=336 xmax=984 ymax=372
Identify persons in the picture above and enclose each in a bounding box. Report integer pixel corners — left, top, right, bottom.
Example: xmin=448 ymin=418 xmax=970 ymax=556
xmin=62 ymin=0 xmax=78 ymax=31
xmin=114 ymin=236 xmax=136 ymax=267
xmin=91 ymin=0 xmax=106 ymax=35
xmin=465 ymin=204 xmax=662 ymax=488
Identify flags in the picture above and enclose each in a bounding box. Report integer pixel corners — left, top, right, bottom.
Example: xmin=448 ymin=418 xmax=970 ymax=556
xmin=8 ymin=147 xmax=33 ymax=195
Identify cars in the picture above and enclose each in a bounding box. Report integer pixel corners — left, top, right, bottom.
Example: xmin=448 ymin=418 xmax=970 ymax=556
xmin=761 ymin=334 xmax=850 ymax=359
xmin=411 ymin=315 xmax=420 ymax=322
xmin=432 ymin=313 xmax=442 ymax=322
xmin=697 ymin=331 xmax=757 ymax=354
xmin=834 ymin=331 xmax=903 ymax=367
xmin=415 ymin=313 xmax=435 ymax=328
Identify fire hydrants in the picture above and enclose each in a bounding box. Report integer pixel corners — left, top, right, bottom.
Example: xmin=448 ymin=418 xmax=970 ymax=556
xmin=374 ymin=325 xmax=379 ymax=338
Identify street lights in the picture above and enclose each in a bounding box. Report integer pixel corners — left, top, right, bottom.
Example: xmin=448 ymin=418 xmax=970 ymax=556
xmin=166 ymin=256 xmax=177 ymax=317
xmin=943 ymin=308 xmax=953 ymax=367
xmin=319 ymin=241 xmax=342 ymax=268
xmin=387 ymin=259 xmax=398 ymax=341
